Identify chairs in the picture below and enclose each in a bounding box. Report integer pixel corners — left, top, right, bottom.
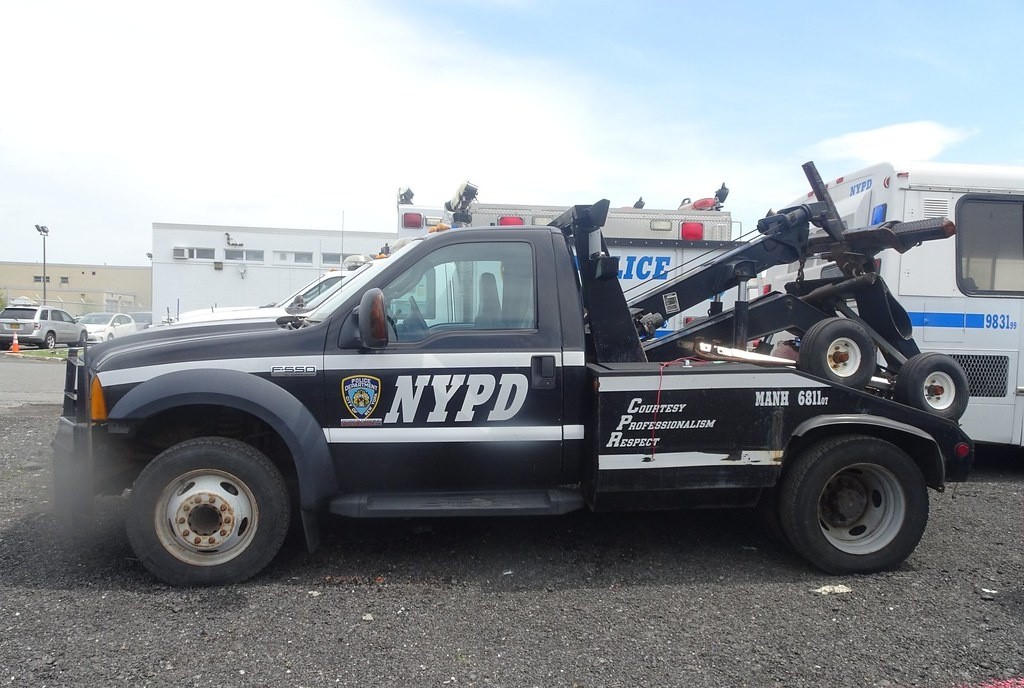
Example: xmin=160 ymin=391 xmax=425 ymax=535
xmin=473 ymin=272 xmax=506 ymax=328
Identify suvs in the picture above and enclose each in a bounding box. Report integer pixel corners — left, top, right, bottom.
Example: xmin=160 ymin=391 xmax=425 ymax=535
xmin=0 ymin=303 xmax=88 ymax=349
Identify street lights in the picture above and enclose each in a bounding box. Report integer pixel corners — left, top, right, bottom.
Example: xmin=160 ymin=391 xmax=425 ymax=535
xmin=36 ymin=224 xmax=48 ymax=306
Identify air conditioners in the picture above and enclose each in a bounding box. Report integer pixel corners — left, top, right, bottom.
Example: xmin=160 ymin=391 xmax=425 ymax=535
xmin=174 ymin=248 xmax=190 ymax=258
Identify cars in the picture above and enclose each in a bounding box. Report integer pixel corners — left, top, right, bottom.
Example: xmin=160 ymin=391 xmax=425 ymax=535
xmin=128 ymin=312 xmax=152 ymax=333
xmin=79 ymin=312 xmax=137 ymax=344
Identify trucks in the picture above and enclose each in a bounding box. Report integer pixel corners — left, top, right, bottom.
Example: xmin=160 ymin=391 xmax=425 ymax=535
xmin=154 ymin=163 xmax=1024 ymax=449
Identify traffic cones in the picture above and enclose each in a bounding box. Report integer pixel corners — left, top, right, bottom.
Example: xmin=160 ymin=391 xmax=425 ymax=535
xmin=12 ymin=331 xmax=19 ymax=353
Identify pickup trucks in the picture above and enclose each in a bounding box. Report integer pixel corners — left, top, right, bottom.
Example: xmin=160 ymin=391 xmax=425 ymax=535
xmin=55 ymin=201 xmax=967 ymax=578
